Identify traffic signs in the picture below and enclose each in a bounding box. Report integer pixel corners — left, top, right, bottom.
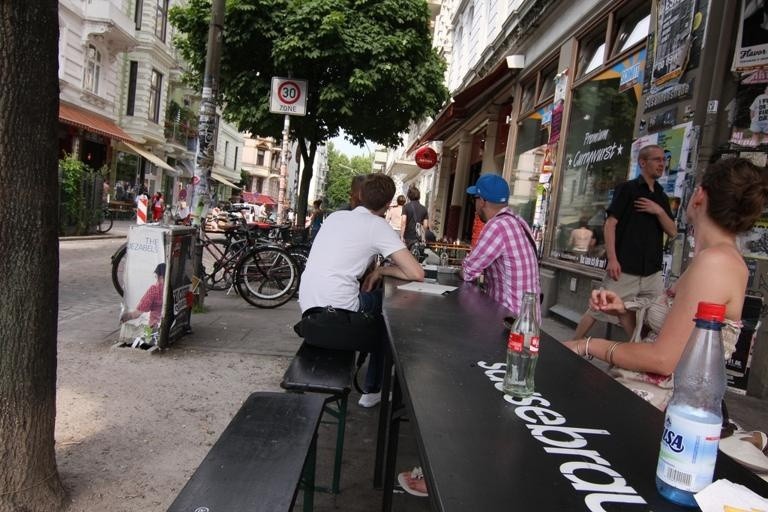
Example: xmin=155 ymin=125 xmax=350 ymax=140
xmin=269 ymin=76 xmax=308 ymax=115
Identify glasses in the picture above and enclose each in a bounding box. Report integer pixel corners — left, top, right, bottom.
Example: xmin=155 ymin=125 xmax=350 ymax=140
xmin=645 ymin=157 xmax=667 ymax=163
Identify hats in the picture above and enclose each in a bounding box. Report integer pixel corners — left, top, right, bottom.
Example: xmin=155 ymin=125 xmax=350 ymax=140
xmin=466 ymin=174 xmax=510 ymax=204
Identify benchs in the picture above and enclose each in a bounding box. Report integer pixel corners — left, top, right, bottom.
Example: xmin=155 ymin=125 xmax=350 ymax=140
xmin=281 ymin=336 xmax=353 ymax=493
xmin=171 ymin=392 xmax=326 ymax=512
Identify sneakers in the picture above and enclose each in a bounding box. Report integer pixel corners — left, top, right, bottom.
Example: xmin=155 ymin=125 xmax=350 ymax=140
xmin=395 ymin=467 xmax=430 ymax=496
xmin=359 ymin=391 xmax=392 ymax=408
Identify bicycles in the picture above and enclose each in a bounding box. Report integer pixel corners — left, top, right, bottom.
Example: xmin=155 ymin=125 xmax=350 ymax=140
xmin=96 ymin=205 xmax=311 ymax=312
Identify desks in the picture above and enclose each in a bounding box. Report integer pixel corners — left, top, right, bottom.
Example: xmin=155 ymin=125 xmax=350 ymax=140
xmin=372 ymin=272 xmax=768 ymax=512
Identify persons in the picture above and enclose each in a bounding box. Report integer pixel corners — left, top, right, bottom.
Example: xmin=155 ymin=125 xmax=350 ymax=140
xmin=566 ymin=217 xmax=593 ymax=257
xmin=294 ymin=171 xmax=437 ymax=408
xmin=103 ymin=179 xmax=165 ymax=223
xmin=460 ymin=173 xmax=543 ymax=327
xmin=567 ymin=144 xmax=678 ymax=340
xmin=120 ymin=263 xmax=174 ymax=341
xmin=397 ymin=155 xmax=767 ymax=498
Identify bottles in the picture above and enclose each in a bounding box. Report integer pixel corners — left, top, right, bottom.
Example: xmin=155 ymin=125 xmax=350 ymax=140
xmin=412 ymin=237 xmax=427 ymax=266
xmin=500 ymin=292 xmax=540 ymax=397
xmin=162 ymin=204 xmax=174 ymax=226
xmin=651 ymin=301 xmax=733 ymax=506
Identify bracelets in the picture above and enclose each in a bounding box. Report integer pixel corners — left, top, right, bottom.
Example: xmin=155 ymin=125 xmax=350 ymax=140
xmin=585 ymin=334 xmax=594 ymax=360
xmin=575 ymin=339 xmax=581 ymax=356
xmin=605 ymin=340 xmax=622 ymax=366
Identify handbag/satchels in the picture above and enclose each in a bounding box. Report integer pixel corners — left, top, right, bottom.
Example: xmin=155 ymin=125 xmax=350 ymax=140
xmin=415 ymin=221 xmax=429 ymax=247
